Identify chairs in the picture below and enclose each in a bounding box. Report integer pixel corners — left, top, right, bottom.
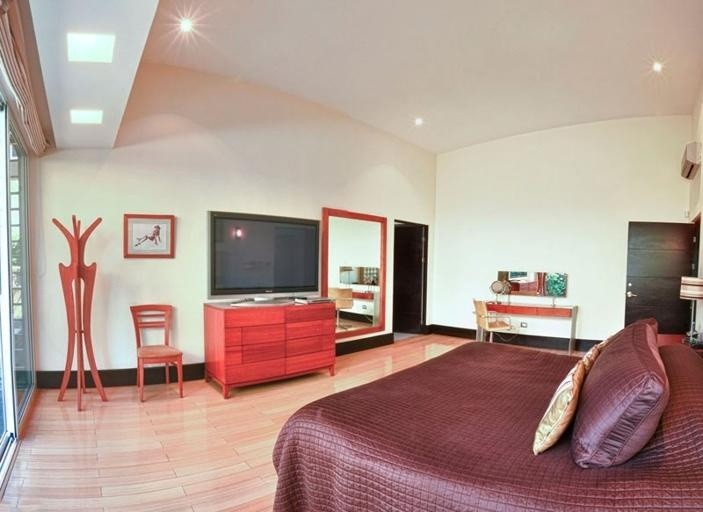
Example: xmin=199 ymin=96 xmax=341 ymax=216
xmin=473 ymin=299 xmax=512 ymax=342
xmin=328 ymin=287 xmax=353 ymax=331
xmin=129 ymin=303 xmax=184 ymax=403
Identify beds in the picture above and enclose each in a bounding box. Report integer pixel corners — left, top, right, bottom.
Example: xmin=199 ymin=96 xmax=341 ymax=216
xmin=275 ymin=340 xmax=703 ymax=511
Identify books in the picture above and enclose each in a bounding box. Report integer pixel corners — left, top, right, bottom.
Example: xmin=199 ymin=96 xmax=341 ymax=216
xmin=295 ymin=297 xmax=332 ymax=304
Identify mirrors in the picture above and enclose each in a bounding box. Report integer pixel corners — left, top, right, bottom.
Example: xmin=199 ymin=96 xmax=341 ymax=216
xmin=321 ymin=207 xmax=387 ymax=338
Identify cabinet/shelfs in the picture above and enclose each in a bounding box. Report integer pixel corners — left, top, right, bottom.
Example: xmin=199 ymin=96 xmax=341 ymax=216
xmin=202 ymin=298 xmax=336 ymax=399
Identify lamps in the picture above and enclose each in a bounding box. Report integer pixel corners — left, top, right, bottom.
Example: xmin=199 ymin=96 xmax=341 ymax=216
xmin=679 ymin=276 xmax=703 ymax=346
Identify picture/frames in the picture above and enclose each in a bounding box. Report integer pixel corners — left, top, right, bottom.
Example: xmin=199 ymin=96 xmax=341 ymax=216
xmin=122 ymin=214 xmax=174 ymax=258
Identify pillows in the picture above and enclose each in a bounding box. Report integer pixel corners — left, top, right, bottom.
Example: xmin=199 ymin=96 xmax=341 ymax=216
xmin=534 ymin=318 xmax=669 ymax=472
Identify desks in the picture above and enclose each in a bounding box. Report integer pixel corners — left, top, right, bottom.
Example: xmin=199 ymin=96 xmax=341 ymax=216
xmin=486 ymin=302 xmax=579 ymax=354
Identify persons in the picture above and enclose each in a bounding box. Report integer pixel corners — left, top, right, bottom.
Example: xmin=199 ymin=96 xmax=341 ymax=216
xmin=134 ymin=225 xmax=162 ymax=247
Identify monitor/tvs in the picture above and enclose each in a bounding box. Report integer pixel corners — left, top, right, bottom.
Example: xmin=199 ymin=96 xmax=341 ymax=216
xmin=209 ymin=209 xmax=320 ymax=307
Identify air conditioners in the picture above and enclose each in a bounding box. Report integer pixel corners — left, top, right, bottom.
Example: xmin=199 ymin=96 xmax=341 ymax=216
xmin=681 ymin=142 xmax=701 ymax=180
